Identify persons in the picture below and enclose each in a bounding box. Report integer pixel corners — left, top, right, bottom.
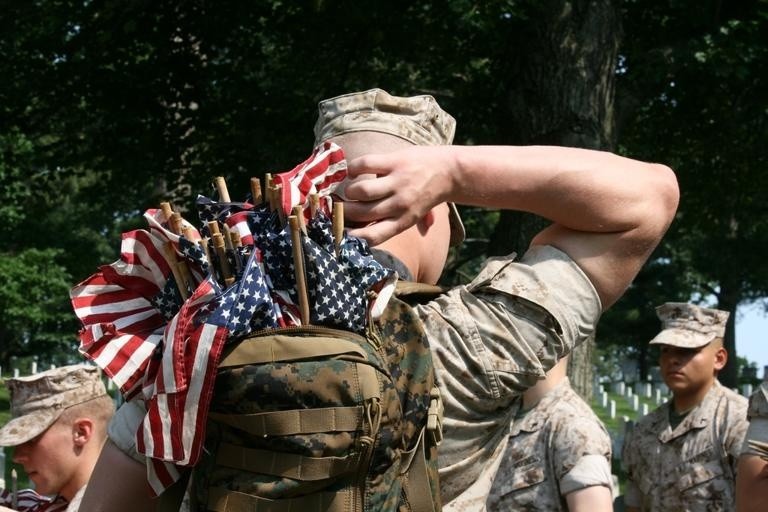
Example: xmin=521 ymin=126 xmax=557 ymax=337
xmin=78 ymin=87 xmax=684 ymax=511
xmin=485 ymin=354 xmax=616 ymax=512
xmin=732 ymin=379 xmax=768 ymax=511
xmin=618 ymin=301 xmax=750 ymax=511
xmin=1 ymin=363 xmax=117 ymax=511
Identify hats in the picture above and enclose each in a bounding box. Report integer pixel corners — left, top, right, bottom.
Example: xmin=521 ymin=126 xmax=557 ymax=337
xmin=649 ymin=303 xmax=730 ymax=348
xmin=0 ymin=363 xmax=109 ymax=447
xmin=314 ymin=87 xmax=466 ymax=247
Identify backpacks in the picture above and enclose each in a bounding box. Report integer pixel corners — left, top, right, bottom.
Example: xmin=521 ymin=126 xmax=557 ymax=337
xmin=178 ymin=280 xmax=442 ymax=511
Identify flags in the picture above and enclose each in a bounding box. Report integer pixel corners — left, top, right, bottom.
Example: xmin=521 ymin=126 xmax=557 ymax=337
xmin=69 ymin=140 xmax=400 ymax=497
